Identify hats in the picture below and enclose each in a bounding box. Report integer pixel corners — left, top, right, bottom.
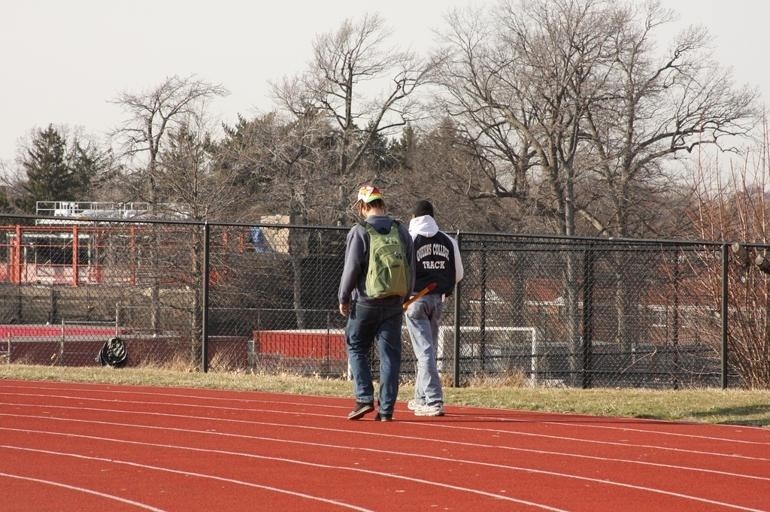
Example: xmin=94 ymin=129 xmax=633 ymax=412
xmin=352 ymin=185 xmax=386 ymax=207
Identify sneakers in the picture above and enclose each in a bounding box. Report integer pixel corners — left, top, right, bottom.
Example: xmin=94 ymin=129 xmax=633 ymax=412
xmin=406 ymin=398 xmax=444 ymax=417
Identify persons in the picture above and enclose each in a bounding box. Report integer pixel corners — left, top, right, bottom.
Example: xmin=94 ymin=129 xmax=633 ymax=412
xmin=404 ymin=200 xmax=464 ymax=418
xmin=337 ymin=186 xmax=416 ymax=423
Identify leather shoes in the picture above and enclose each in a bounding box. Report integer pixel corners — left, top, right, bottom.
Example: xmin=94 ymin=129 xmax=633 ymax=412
xmin=376 ymin=411 xmax=394 ymax=422
xmin=346 ymin=403 xmax=373 ymax=420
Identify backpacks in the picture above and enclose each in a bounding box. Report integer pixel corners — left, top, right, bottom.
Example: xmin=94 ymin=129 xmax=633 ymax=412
xmin=352 ymin=218 xmax=414 ymax=302
